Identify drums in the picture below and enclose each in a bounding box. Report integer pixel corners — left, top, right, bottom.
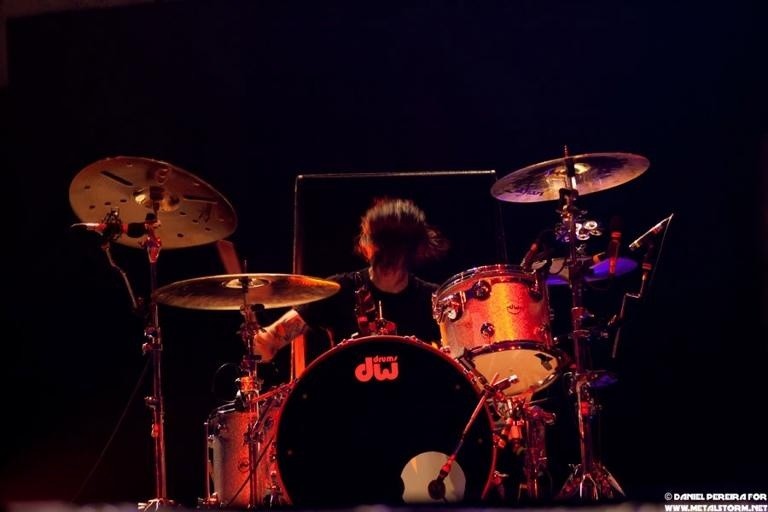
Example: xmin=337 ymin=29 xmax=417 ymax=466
xmin=211 ymin=401 xmax=282 ymax=506
xmin=274 ymin=336 xmax=498 ymax=512
xmin=433 ymin=263 xmax=561 ymax=397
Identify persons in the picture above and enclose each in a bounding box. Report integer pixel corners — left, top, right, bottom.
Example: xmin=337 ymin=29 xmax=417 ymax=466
xmin=250 ymin=201 xmax=446 ymax=364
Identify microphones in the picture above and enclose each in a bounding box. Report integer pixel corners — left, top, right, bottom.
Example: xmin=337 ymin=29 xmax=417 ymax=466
xmin=624 ymin=217 xmax=669 ymax=252
xmin=72 ymin=222 xmax=147 ymax=239
xmin=521 ymin=239 xmax=536 ymax=270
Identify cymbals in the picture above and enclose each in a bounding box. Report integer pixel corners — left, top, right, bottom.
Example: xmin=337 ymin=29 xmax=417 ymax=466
xmin=529 ymin=253 xmax=642 ymax=289
xmin=154 ymin=273 xmax=340 ymax=310
xmin=69 ymin=159 xmax=238 ymax=249
xmin=491 ymin=153 xmax=650 ymax=201
